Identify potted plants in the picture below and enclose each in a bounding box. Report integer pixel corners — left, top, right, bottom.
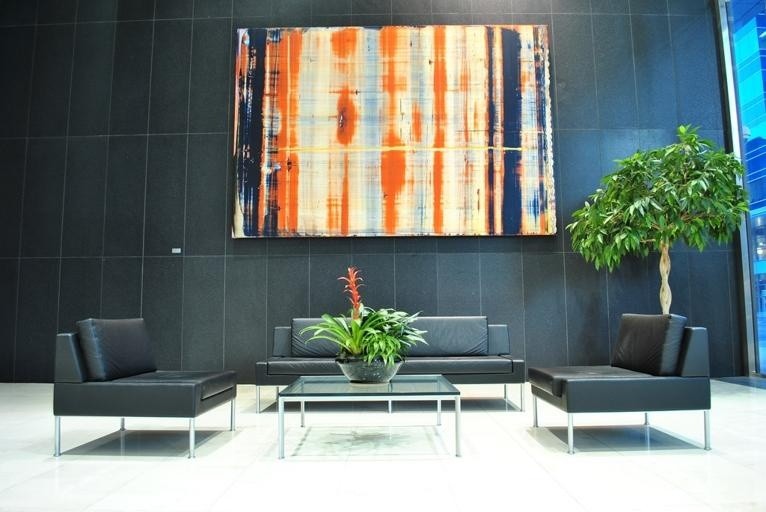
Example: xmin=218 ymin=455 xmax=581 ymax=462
xmin=298 ymin=267 xmax=430 ymax=386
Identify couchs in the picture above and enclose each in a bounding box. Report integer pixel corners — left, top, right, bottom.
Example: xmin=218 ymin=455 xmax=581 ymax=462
xmin=254 ymin=316 xmax=528 ymax=412
xmin=528 ymin=313 xmax=713 ymax=454
xmin=52 ymin=317 xmax=237 ymax=459
